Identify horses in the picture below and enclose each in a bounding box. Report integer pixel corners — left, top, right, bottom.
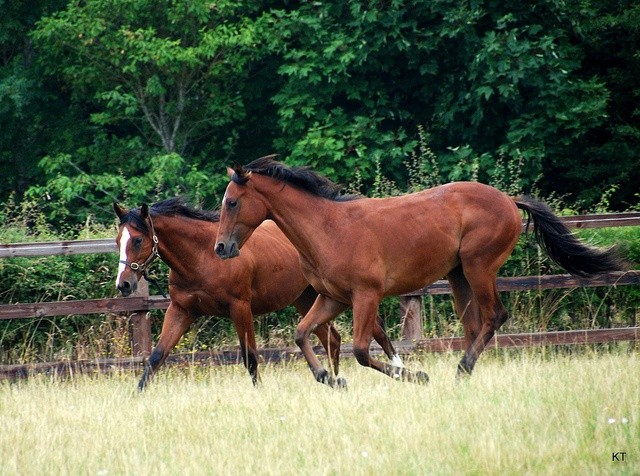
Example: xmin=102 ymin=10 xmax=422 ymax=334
xmin=214 ymin=153 xmax=632 ymax=393
xmin=113 ymin=194 xmax=405 ymax=393
